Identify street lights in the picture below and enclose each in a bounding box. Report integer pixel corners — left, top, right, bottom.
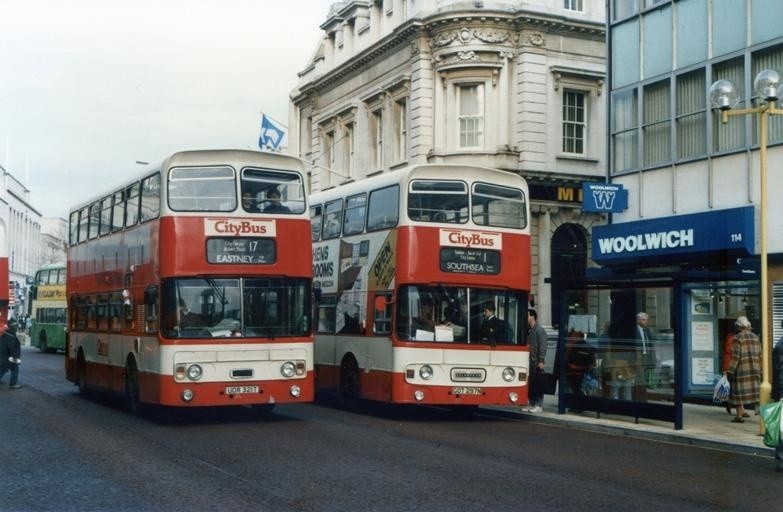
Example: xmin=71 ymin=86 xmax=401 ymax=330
xmin=709 ymin=69 xmax=783 ymax=436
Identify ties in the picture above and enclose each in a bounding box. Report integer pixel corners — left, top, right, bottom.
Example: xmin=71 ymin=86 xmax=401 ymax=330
xmin=641 ymin=328 xmax=649 ymax=355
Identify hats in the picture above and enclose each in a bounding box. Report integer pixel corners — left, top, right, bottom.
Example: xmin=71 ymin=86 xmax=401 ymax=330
xmin=733 ymin=314 xmax=751 ymax=328
xmin=482 ymin=303 xmax=496 ymax=312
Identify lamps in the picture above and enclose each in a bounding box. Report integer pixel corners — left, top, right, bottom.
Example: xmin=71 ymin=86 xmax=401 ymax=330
xmin=709 ymin=288 xmax=751 ymax=304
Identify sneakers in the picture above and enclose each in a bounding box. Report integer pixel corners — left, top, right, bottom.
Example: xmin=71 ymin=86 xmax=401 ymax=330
xmin=731 ymin=410 xmax=751 ymax=424
xmin=519 ymin=405 xmax=543 ymax=414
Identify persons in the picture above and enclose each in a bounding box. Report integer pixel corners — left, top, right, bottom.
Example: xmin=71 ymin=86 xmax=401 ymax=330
xmin=562 ymin=306 xmax=658 ymax=416
xmin=396 ymin=295 xmax=514 ymax=346
xmin=724 ymin=332 xmax=760 ymax=417
xmin=723 ymin=315 xmax=761 ymax=425
xmin=262 ymin=189 xmax=292 ymax=214
xmin=0 ymin=317 xmax=21 ymax=388
xmin=165 ymin=292 xmax=204 ymax=330
xmin=518 ymin=309 xmax=548 ymax=414
xmin=772 ymin=317 xmax=782 ymax=473
xmin=8 ymin=313 xmax=31 ymax=335
xmin=240 ymin=189 xmax=262 ymax=213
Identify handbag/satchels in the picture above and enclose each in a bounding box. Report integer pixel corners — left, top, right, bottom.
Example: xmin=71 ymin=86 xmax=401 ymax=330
xmin=760 ymin=398 xmax=783 ymax=448
xmin=529 ymin=368 xmax=557 ymax=396
xmin=8 ymin=356 xmax=22 ymax=365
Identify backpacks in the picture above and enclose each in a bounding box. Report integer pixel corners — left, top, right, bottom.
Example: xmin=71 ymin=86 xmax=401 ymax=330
xmin=569 ymin=335 xmax=596 ymax=370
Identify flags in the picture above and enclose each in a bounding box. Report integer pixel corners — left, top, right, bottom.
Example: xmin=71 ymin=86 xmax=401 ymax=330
xmin=256 ymin=115 xmax=285 ymax=151
